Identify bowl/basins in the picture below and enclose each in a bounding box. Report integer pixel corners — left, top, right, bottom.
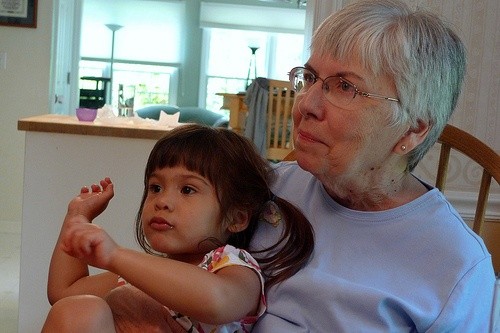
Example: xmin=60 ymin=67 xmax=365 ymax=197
xmin=76 ymin=107 xmax=97 ymax=121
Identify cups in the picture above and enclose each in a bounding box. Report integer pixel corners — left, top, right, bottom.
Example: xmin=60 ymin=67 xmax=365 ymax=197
xmin=118 ymin=84 xmax=136 ymax=117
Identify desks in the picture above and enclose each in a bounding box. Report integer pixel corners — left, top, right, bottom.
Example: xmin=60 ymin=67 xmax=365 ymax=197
xmin=216 ymin=92 xmax=295 ymax=130
xmin=18 ymin=112 xmax=179 ymax=333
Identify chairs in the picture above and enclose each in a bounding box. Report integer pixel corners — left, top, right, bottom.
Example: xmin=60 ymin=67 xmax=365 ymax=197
xmin=284 ymin=122 xmax=500 ymax=237
xmin=267 ymin=79 xmax=294 ymax=161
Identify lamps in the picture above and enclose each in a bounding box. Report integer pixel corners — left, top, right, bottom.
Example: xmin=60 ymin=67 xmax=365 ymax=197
xmin=105 ymin=23 xmax=123 ymax=104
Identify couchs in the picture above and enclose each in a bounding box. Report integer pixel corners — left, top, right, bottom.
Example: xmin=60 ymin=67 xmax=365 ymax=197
xmin=137 ymin=105 xmax=230 ymax=127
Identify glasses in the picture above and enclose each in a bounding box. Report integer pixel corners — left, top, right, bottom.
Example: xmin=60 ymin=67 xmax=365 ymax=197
xmin=286 ymin=66 xmax=401 ymax=109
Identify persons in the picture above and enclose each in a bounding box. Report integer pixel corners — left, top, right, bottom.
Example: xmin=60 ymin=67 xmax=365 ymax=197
xmin=105 ymin=0 xmax=500 ymax=333
xmin=41 ymin=125 xmax=315 ymax=333
